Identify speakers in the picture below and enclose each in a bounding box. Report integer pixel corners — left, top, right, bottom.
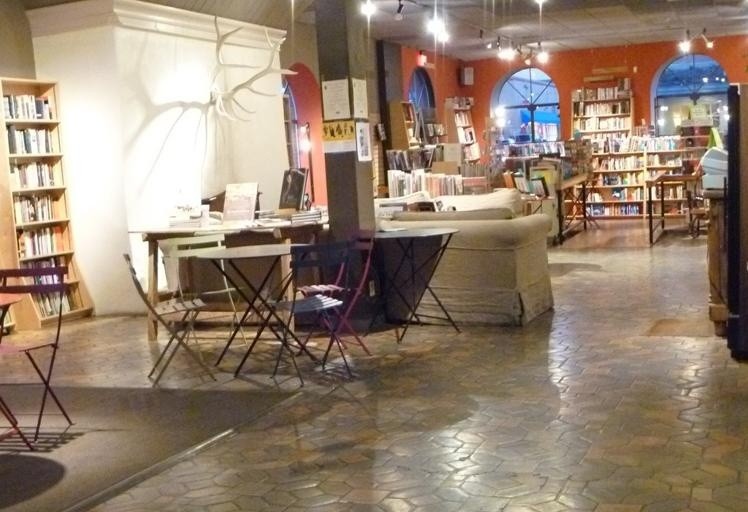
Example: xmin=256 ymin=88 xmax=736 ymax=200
xmin=460 ymin=67 xmax=475 ymax=87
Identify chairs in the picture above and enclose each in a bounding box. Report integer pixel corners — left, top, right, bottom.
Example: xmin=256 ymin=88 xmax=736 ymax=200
xmin=265 ymin=238 xmax=355 ymax=388
xmin=293 ymin=226 xmax=376 ymax=365
xmin=0 ymin=264 xmax=73 ymax=451
xmin=122 ymin=251 xmax=219 ymax=389
xmin=158 ymin=233 xmax=248 ymax=351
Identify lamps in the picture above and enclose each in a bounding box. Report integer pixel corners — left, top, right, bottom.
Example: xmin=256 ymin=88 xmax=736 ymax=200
xmin=359 ymin=0 xmax=549 ymax=66
xmin=699 ymin=29 xmax=715 ymax=51
xmin=677 ymin=25 xmax=695 ymax=53
xmin=297 ymin=122 xmax=314 ymax=204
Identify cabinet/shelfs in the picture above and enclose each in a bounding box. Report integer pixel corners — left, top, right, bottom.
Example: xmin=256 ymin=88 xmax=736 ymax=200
xmin=557 ymin=83 xmax=710 ymax=246
xmin=0 ymin=74 xmax=97 ymax=332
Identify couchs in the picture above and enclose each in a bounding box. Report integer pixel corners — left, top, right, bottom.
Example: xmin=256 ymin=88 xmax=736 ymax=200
xmin=376 ymin=205 xmax=551 ymax=325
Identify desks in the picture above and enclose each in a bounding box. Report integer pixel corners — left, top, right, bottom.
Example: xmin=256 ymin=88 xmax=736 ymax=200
xmin=362 ymin=225 xmax=462 ymax=345
xmin=193 ymin=241 xmax=324 ymax=378
xmin=126 ymin=212 xmax=328 ymax=310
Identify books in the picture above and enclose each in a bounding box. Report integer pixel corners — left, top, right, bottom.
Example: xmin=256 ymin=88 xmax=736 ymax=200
xmin=571 ymin=78 xmax=685 ymax=215
xmin=385 ymin=145 xmax=492 ymax=198
xmin=453 ymin=97 xmax=481 ymax=164
xmin=406 ymin=106 xmax=422 ymax=146
xmin=502 ymin=122 xmax=563 ymax=197
xmin=1 ymin=94 xmax=73 ymax=324
xmin=290 ymin=212 xmax=322 ymax=224
